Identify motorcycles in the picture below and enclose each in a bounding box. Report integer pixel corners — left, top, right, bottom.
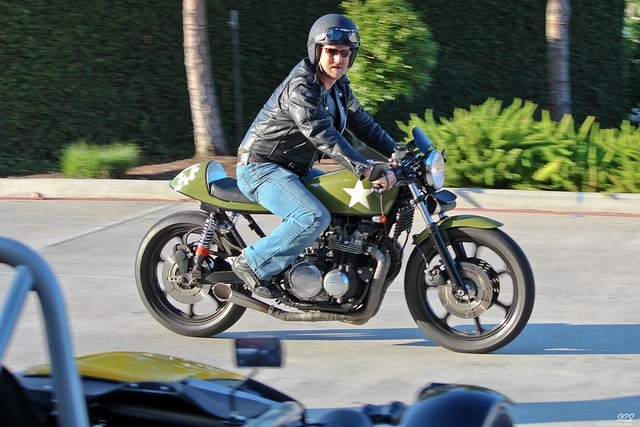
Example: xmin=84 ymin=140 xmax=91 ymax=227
xmin=135 ymin=127 xmax=536 ymax=355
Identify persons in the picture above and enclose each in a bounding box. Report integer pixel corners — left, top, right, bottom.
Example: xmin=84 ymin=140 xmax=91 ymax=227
xmin=232 ymin=13 xmax=407 ymax=299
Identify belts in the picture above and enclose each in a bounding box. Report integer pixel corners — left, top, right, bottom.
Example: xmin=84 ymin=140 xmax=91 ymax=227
xmin=236 ymin=150 xmax=266 ymax=164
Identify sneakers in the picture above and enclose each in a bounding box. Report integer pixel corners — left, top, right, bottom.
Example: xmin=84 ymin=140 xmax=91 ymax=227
xmin=231 ymin=253 xmax=282 ymax=298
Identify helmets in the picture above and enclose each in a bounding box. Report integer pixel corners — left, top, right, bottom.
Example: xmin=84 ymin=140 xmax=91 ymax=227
xmin=307 ymin=13 xmax=361 ymax=69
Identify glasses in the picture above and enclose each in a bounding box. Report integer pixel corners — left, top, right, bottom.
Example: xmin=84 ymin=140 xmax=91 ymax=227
xmin=321 ymin=46 xmax=352 ymax=57
xmin=307 ymin=25 xmax=361 ymax=46
